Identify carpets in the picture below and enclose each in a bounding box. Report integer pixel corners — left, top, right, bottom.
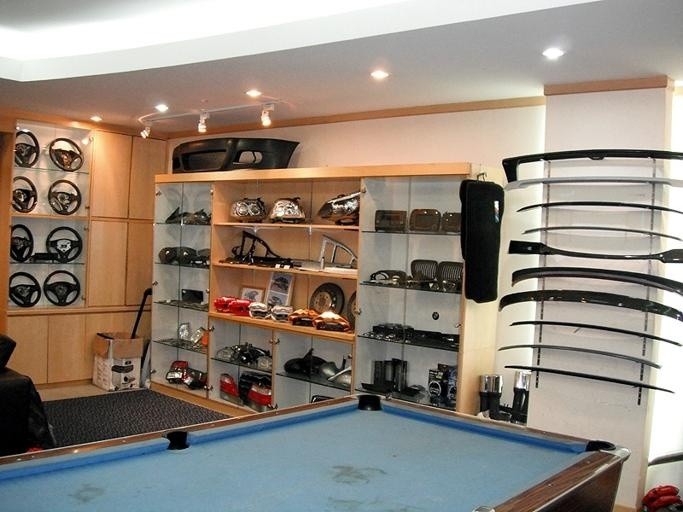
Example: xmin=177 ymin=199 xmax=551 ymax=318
xmin=38 ymin=385 xmax=229 ymax=449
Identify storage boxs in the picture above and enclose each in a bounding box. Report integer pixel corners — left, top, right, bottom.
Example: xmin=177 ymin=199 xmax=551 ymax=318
xmin=89 ymin=331 xmax=142 ymax=396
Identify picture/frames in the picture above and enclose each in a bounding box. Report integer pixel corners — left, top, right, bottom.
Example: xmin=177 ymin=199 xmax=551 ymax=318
xmin=236 ymin=283 xmax=266 ymax=304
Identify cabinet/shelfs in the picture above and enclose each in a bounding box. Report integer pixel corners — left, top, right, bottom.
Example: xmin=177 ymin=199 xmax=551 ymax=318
xmin=90 ymin=129 xmax=166 ymax=222
xmin=89 ymin=215 xmax=155 ymax=307
xmin=84 ymin=309 xmax=153 ymax=385
xmin=8 ymin=314 xmax=84 ymax=387
xmin=152 ymin=162 xmax=508 ymax=421
xmin=13 ymin=121 xmax=87 ymax=311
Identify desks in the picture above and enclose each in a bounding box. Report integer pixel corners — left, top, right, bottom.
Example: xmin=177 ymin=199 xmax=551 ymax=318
xmin=0 ymin=392 xmax=631 ymax=511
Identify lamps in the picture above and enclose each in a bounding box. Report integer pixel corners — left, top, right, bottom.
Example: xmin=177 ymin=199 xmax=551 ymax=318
xmin=138 ymin=101 xmax=272 ymax=140
